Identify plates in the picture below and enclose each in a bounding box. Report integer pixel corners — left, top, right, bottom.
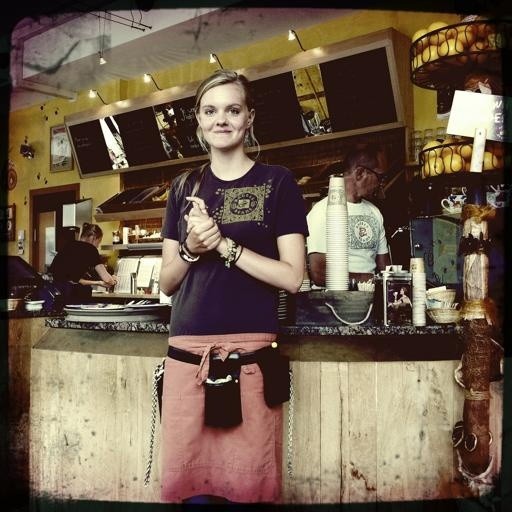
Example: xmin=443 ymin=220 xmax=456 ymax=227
xmin=126 ymin=303 xmax=171 ymax=308
xmin=81 ymin=304 xmax=124 ymax=311
xmin=277 ymin=277 xmax=312 ymax=320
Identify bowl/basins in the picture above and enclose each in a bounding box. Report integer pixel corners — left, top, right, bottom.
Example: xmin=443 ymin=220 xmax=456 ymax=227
xmin=304 ymin=287 xmax=375 ymax=327
xmin=426 ymin=305 xmax=461 ymax=325
xmin=8 ymin=299 xmax=23 ymax=311
xmin=426 ymin=289 xmax=456 ymax=310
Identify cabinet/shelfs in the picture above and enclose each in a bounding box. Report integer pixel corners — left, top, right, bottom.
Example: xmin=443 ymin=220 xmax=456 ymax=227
xmin=91 ymin=242 xmax=163 ymax=299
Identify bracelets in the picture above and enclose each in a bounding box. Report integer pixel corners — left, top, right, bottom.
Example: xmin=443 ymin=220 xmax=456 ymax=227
xmin=178 ymin=237 xmax=201 ymax=265
xmin=220 ymin=236 xmax=244 ymax=269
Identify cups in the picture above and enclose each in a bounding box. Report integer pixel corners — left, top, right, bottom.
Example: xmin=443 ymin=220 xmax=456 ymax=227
xmin=410 ymin=257 xmax=427 ymax=327
xmin=326 ymin=177 xmax=350 ymax=292
xmin=440 ymin=194 xmax=465 ymax=215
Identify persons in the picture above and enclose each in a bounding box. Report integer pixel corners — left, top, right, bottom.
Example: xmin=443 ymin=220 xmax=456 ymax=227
xmin=50 ymin=221 xmax=118 ymax=289
xmin=387 ymin=286 xmax=412 ymax=321
xmin=302 ymin=144 xmax=393 ymax=288
xmin=154 ymin=68 xmax=310 ymax=504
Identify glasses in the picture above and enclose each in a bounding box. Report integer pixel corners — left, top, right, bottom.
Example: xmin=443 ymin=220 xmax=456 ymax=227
xmin=356 ymin=164 xmax=388 ymax=183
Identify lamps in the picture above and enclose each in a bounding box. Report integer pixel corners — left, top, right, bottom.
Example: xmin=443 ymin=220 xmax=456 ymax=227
xmin=209 ymin=52 xmax=224 ymax=71
xmin=142 ymin=72 xmax=161 ymax=92
xmin=96 ymin=10 xmax=108 ymax=66
xmin=87 ymin=89 xmax=108 ymax=105
xmin=287 ymin=28 xmax=305 ymax=50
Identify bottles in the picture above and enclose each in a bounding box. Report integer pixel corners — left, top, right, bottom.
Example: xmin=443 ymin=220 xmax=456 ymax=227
xmin=135 ymin=225 xmax=142 ymax=241
xmin=112 ymin=230 xmax=121 ymax=245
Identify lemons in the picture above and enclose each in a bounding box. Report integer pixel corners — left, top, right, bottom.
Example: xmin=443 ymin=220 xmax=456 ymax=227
xmin=410 ymin=15 xmax=495 ymax=72
xmin=421 ymin=139 xmax=504 ymax=180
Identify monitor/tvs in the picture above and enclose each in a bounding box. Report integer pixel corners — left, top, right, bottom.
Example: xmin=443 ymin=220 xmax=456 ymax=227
xmin=407 ymin=215 xmax=463 ymax=299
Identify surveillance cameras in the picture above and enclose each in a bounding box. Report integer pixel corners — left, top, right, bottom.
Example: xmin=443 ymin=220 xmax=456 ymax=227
xmin=20 ymin=143 xmax=34 ymax=159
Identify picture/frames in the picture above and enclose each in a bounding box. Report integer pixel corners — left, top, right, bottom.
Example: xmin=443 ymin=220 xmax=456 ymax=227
xmin=48 ymin=125 xmax=76 ymax=174
xmin=6 ymin=204 xmax=16 ymax=243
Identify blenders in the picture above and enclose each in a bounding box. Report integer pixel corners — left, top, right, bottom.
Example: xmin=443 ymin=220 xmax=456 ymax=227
xmin=303 ymin=110 xmax=326 ymax=136
